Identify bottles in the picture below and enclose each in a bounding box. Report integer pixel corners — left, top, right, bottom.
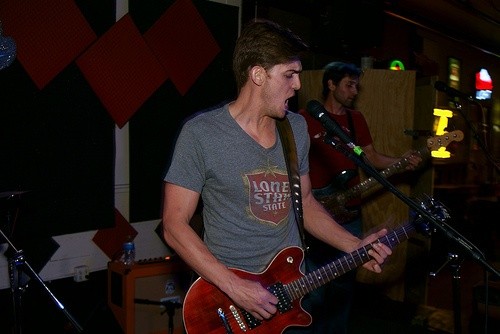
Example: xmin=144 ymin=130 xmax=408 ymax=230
xmin=123 ymin=234 xmax=135 ymax=265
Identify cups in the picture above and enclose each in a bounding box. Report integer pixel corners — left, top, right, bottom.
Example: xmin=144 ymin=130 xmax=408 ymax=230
xmin=361 ymin=56 xmax=374 ymax=71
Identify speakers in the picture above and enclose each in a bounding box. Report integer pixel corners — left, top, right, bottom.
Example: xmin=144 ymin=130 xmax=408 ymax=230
xmin=105 ymin=257 xmax=203 ymax=334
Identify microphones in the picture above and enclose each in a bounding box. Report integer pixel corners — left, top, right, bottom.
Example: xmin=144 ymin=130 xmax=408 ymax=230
xmin=433 ymin=80 xmax=476 ymax=102
xmin=306 ymin=99 xmax=362 ymax=155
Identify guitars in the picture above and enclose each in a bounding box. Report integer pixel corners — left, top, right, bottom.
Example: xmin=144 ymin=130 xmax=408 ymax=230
xmin=310 ymin=128 xmax=465 ymax=222
xmin=182 ymin=191 xmax=452 ymax=334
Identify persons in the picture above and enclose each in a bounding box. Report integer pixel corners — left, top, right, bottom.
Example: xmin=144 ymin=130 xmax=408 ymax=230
xmin=164 ymin=22 xmax=393 ymax=334
xmin=298 ymin=64 xmax=423 ymax=334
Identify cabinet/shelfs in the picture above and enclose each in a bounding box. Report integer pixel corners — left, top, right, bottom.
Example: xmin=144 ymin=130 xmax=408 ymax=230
xmin=410 ymin=74 xmax=481 ymax=255
